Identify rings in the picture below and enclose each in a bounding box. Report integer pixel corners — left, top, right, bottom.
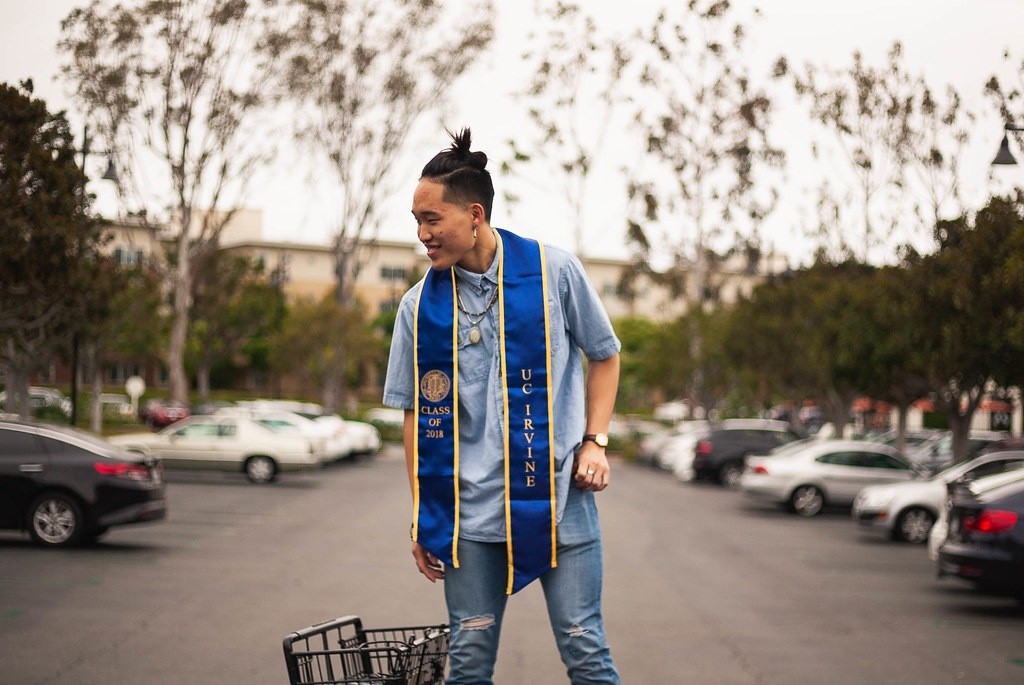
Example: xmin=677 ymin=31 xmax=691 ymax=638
xmin=587 ymin=469 xmax=594 ymax=476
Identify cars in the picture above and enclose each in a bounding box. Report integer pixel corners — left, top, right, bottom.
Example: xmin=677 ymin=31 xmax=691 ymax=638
xmin=106 ymin=414 xmax=323 ymax=484
xmin=200 ymin=398 xmax=384 ymax=466
xmin=140 ymin=397 xmax=191 ymax=427
xmin=1 ymin=386 xmax=71 ymax=420
xmin=609 ymin=406 xmax=1024 ymax=599
xmin=101 ymin=392 xmax=137 ymax=425
xmin=1 ymin=413 xmax=166 ymax=546
xmin=364 ymin=410 xmax=403 ymax=434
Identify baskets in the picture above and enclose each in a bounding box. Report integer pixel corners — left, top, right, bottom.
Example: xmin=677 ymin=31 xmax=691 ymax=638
xmin=284 ymin=616 xmax=456 ymax=685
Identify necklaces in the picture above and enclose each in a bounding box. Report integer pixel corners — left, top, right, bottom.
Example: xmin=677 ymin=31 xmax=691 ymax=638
xmin=455 ymin=277 xmax=499 ymax=343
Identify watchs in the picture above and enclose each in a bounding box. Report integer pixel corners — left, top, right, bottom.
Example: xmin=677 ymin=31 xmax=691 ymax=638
xmin=582 ymin=433 xmax=609 ymax=447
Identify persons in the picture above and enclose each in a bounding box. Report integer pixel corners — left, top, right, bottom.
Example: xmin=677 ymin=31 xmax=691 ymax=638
xmin=382 ymin=126 xmax=623 ymax=685
